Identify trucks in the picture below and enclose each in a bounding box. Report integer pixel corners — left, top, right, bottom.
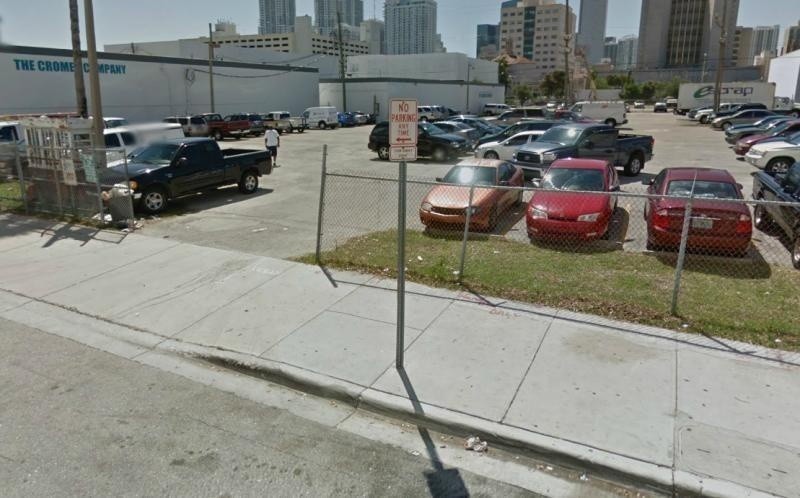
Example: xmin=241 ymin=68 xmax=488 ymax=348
xmin=676 ymin=81 xmax=794 ymax=114
xmin=570 ymin=101 xmax=627 ymax=128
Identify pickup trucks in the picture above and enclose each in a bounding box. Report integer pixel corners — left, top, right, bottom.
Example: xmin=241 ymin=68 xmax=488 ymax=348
xmin=106 ymin=136 xmax=271 ymax=215
xmin=512 ymin=122 xmax=655 ymax=186
xmin=749 ymin=158 xmax=800 ymax=268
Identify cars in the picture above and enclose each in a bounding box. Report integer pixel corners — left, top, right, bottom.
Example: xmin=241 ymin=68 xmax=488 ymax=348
xmin=417 ymin=99 xmax=605 ymax=150
xmin=164 ymin=109 xmax=308 ymax=140
xmin=688 ymin=102 xmax=800 ymax=172
xmin=0 ymin=114 xmax=129 ymax=175
xmin=418 ymin=156 xmax=526 ymax=235
xmin=634 ymin=100 xmax=645 ymax=108
xmin=643 ymin=165 xmax=754 ymax=260
xmin=666 ymin=98 xmax=676 ymax=109
xmin=474 ymin=130 xmax=547 ymax=163
xmin=653 ymin=101 xmax=668 ymax=112
xmin=336 ymin=111 xmax=377 ymax=127
xmin=521 ymin=155 xmax=621 ymax=244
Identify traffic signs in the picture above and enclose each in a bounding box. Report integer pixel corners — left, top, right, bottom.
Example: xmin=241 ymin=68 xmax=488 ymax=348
xmin=387 ymin=96 xmax=419 ymax=147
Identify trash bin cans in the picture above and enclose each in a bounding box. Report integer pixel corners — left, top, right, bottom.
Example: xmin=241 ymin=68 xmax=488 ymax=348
xmin=108 ymin=194 xmax=135 ymax=222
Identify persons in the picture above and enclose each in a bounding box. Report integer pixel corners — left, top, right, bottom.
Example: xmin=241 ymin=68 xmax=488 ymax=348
xmin=264 ymin=125 xmax=280 ymax=167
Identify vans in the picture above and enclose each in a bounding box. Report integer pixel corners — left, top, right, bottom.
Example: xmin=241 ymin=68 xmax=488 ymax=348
xmin=98 ymin=122 xmax=187 ymax=164
xmin=300 ymin=105 xmax=339 ymax=130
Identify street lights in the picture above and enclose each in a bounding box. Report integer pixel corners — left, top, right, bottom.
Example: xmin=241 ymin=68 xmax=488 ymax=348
xmin=702 ymin=52 xmax=707 ymax=84
xmin=561 ymin=0 xmax=573 ymax=102
xmin=713 ymin=0 xmax=729 ymax=113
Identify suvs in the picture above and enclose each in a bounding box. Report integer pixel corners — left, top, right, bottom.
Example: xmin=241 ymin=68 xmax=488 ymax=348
xmin=366 ymin=119 xmax=468 ymax=164
